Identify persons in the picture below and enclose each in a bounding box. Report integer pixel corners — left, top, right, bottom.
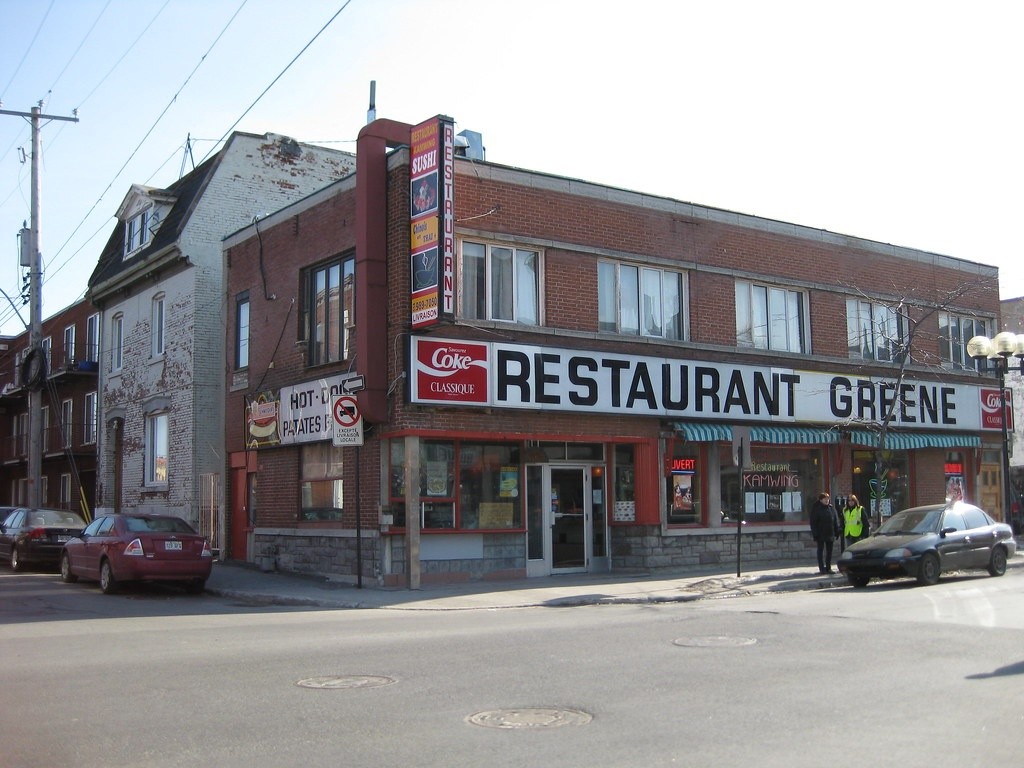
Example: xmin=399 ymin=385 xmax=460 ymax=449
xmin=841 ymin=494 xmax=871 ymax=546
xmin=810 ymin=493 xmax=840 ymax=574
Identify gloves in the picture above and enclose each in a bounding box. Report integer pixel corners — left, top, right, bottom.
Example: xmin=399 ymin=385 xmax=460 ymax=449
xmin=812 ymin=536 xmax=817 ymax=541
xmin=835 ymin=533 xmax=839 ymax=540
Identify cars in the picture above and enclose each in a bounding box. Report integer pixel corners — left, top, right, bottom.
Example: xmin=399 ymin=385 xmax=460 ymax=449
xmin=59 ymin=513 xmax=213 ymax=594
xmin=836 ymin=500 xmax=1017 ymax=589
xmin=0 ymin=506 xmax=87 ymax=573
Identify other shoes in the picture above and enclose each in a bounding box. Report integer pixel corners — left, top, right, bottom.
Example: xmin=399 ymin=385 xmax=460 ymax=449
xmin=820 ymin=567 xmax=830 ymax=574
xmin=826 ymin=566 xmax=836 ymax=574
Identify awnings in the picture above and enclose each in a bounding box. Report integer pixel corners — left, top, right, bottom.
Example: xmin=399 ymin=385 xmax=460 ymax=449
xmin=676 ymin=422 xmax=843 ymax=448
xmin=846 ymin=428 xmax=984 ymax=450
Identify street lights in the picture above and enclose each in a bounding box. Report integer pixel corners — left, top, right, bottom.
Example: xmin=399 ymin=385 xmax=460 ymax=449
xmin=966 ymin=331 xmax=1024 ymax=524
